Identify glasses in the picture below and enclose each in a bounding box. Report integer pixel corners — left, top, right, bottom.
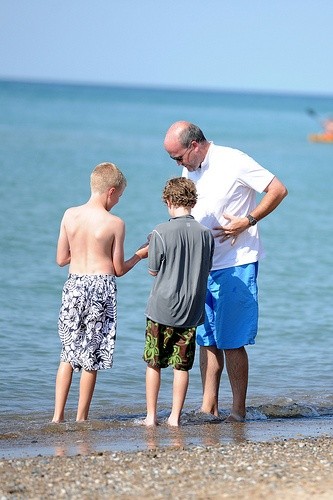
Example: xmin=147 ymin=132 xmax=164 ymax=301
xmin=169 ymin=141 xmax=191 ymax=161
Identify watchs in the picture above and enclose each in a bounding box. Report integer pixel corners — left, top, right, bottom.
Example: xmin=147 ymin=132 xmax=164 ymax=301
xmin=246 ymin=214 xmax=258 ymax=227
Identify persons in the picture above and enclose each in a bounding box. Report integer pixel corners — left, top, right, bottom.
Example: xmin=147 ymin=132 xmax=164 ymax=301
xmin=163 ymin=120 xmax=288 ymax=422
xmin=51 ymin=161 xmax=149 ymax=423
xmin=133 ymin=177 xmax=215 ymax=427
